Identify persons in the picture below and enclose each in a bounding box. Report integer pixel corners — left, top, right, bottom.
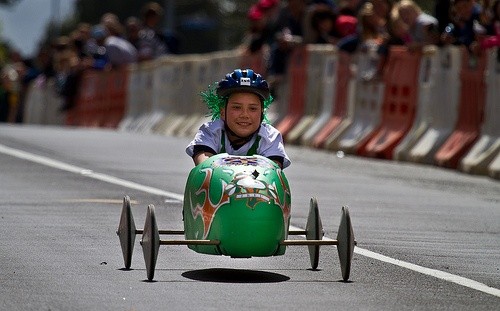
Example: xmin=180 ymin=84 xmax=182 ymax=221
xmin=0 ymin=0 xmax=500 ymax=123
xmin=184 ymin=69 xmax=291 ymax=170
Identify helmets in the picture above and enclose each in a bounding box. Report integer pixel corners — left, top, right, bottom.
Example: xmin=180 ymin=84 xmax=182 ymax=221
xmin=216 ymin=69 xmax=269 ymax=101
